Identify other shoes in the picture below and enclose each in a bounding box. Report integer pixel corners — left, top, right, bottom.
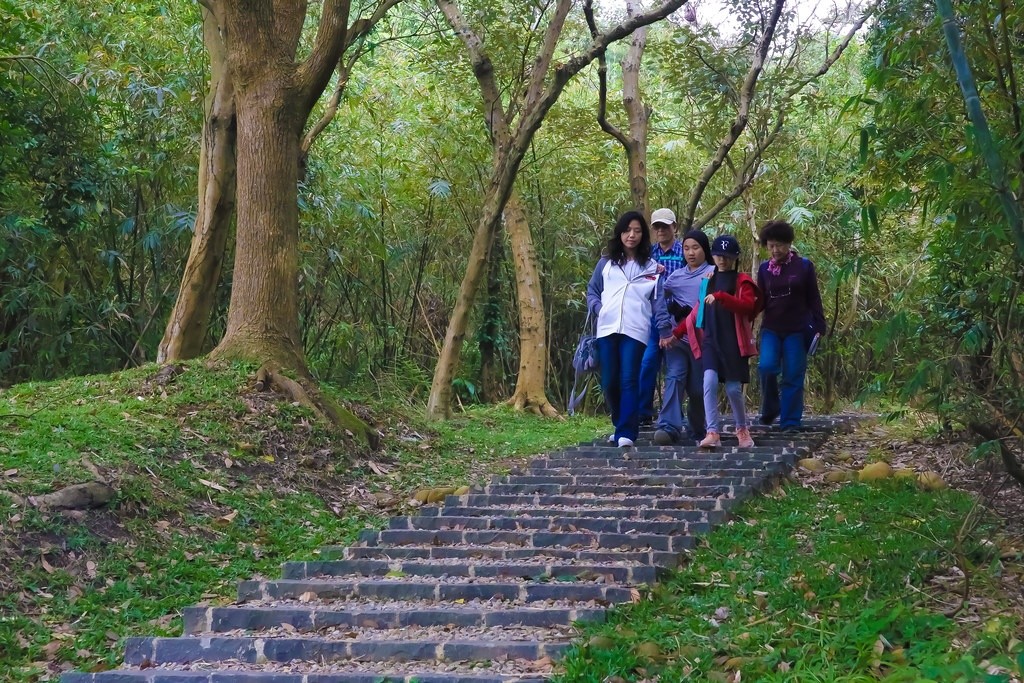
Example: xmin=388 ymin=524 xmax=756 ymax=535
xmin=638 ymin=417 xmax=652 ymax=427
xmin=760 ymin=409 xmax=780 ymax=427
xmin=785 ymin=425 xmax=799 ymax=434
xmin=654 ymin=430 xmax=675 ymax=444
xmin=619 ymin=437 xmax=632 ymax=447
xmin=609 ymin=434 xmax=615 ymax=442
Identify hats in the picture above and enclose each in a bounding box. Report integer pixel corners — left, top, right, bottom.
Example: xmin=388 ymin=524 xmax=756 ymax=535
xmin=650 ymin=207 xmax=676 ymax=225
xmin=710 ymin=235 xmax=741 ymax=259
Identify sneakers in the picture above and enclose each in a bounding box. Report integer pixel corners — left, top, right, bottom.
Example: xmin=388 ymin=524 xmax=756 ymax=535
xmin=736 ymin=426 xmax=754 ymax=448
xmin=701 ymin=430 xmax=722 ymax=447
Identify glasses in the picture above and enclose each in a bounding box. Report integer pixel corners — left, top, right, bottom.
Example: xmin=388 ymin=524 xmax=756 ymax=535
xmin=652 ymin=224 xmax=671 ymax=230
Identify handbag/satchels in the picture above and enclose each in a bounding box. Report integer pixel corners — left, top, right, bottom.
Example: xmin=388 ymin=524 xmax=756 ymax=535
xmin=573 ymin=298 xmax=600 ymax=371
xmin=803 ymin=320 xmax=822 ymax=356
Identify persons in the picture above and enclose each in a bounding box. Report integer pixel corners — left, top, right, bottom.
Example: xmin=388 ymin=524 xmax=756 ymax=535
xmin=587 ymin=208 xmax=826 ymax=449
